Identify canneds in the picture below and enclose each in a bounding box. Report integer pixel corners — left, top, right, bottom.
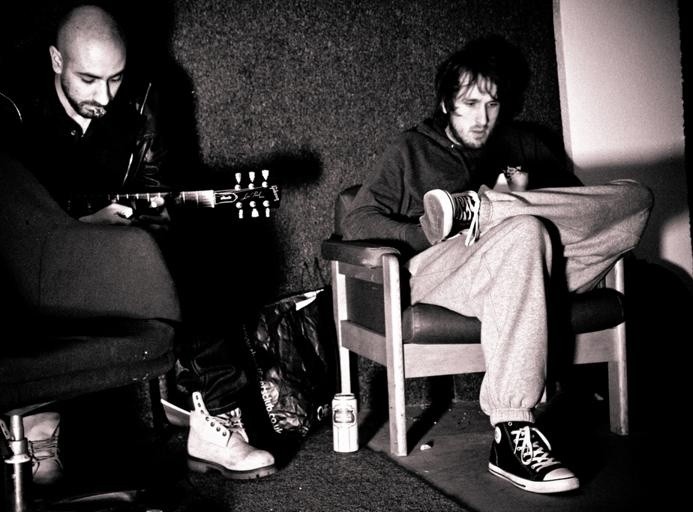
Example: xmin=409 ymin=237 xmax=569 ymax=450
xmin=332 ymin=393 xmax=359 ymax=453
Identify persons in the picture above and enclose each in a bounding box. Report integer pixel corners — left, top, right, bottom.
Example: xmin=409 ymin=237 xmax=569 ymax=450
xmin=338 ymin=46 xmax=656 ymax=496
xmin=0 ymin=2 xmax=276 ymax=492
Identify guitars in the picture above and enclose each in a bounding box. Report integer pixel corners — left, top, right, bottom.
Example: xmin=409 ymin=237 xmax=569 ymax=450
xmin=51 ymin=169 xmax=280 ymax=219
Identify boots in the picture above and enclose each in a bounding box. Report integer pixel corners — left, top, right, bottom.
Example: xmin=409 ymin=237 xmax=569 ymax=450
xmin=486 ymin=419 xmax=581 ymax=493
xmin=417 ymin=187 xmax=481 ymax=246
xmin=185 ymin=391 xmax=277 ymax=481
xmin=10 ymin=411 xmax=79 ymax=508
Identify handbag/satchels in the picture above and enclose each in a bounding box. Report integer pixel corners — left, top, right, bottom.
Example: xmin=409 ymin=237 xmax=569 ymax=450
xmin=238 ymin=287 xmax=340 ymax=440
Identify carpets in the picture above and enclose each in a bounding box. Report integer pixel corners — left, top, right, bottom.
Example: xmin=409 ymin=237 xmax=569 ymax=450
xmin=0 ymin=382 xmax=472 ymax=512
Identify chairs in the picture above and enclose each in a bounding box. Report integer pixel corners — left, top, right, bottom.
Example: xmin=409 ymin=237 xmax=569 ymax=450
xmin=0 ymin=151 xmax=179 ymax=457
xmin=322 ymin=189 xmax=637 ymax=457
